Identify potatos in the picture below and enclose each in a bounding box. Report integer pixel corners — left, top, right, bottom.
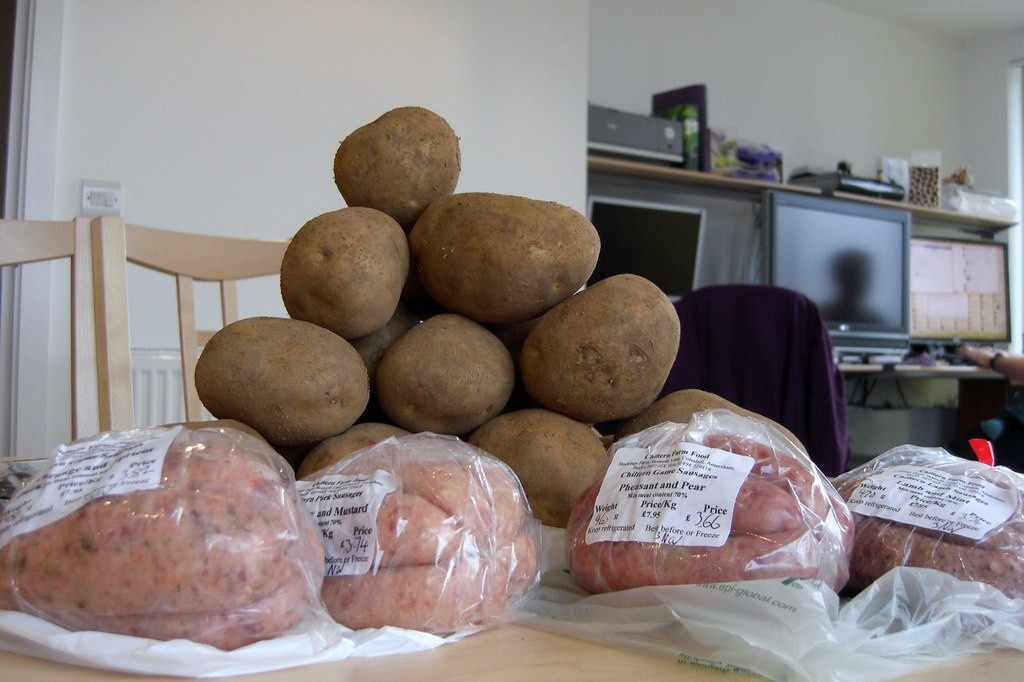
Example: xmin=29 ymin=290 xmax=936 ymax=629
xmin=193 ymin=105 xmax=810 ymax=532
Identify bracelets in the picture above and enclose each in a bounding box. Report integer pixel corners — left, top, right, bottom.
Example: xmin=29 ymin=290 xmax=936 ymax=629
xmin=990 ymin=352 xmax=1006 ymax=369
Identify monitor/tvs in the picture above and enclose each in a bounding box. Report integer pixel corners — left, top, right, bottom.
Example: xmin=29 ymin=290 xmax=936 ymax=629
xmin=585 ymin=196 xmax=708 ymax=302
xmin=909 ymin=234 xmax=1013 ymax=358
xmin=761 ymin=189 xmax=912 ymax=362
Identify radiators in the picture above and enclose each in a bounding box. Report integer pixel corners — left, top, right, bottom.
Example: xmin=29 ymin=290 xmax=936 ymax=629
xmin=127 ymin=347 xmax=218 ymax=426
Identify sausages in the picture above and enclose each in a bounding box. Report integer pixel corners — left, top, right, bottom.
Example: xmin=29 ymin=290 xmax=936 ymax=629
xmin=0 ymin=432 xmax=1024 ymax=649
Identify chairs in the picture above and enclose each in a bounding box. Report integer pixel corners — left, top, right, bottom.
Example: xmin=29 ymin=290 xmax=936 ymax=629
xmin=2 ymin=218 xmax=293 ymax=477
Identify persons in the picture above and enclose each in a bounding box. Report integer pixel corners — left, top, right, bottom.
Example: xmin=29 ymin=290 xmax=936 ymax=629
xmin=960 ymin=344 xmax=1024 ymax=474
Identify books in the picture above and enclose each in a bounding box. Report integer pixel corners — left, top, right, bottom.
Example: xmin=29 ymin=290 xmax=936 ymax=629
xmin=649 ymin=104 xmax=699 ymax=170
xmin=652 ymin=84 xmax=710 ymax=173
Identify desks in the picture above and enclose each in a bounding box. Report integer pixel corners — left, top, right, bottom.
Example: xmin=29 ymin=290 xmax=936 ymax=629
xmin=837 ymin=363 xmax=1012 ymax=457
